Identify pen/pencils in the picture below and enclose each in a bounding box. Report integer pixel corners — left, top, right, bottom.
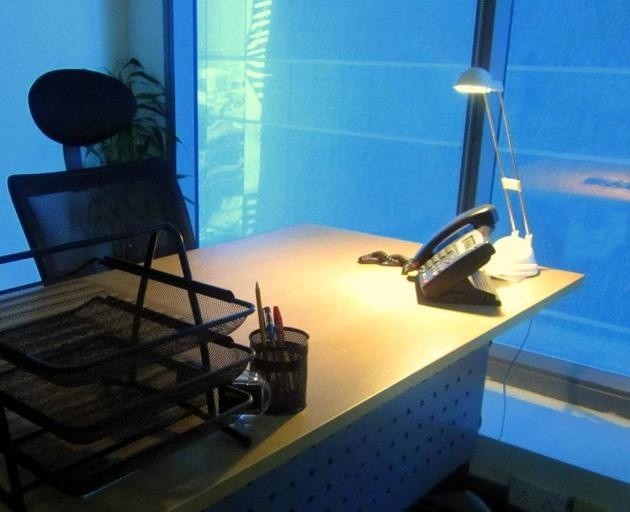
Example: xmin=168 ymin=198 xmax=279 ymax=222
xmin=255 ymin=281 xmax=294 ymax=403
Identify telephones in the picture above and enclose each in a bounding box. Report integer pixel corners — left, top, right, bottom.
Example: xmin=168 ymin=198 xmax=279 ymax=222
xmin=413 ymin=204 xmax=503 ymax=306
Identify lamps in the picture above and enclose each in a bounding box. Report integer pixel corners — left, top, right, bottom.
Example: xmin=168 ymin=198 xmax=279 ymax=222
xmin=454 ymin=67 xmax=540 ymax=280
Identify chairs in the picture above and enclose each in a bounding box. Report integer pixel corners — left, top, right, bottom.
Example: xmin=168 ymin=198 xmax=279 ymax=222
xmin=7 ymin=70 xmax=196 ymax=285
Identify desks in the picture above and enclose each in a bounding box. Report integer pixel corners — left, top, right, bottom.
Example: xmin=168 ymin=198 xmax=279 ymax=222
xmin=0 ymin=222 xmax=585 ymax=511
